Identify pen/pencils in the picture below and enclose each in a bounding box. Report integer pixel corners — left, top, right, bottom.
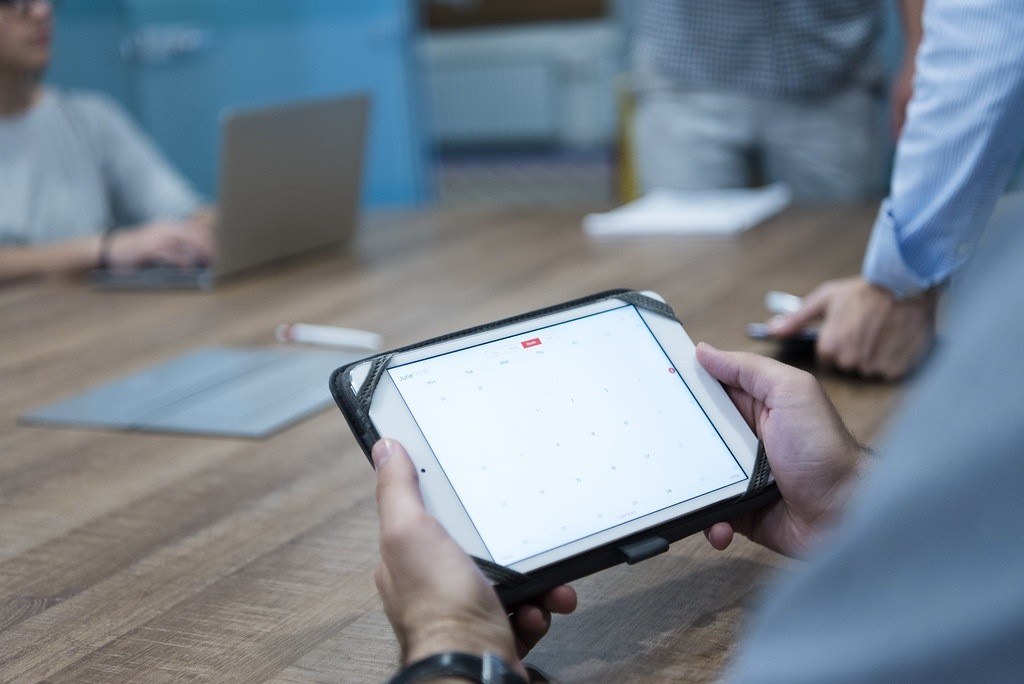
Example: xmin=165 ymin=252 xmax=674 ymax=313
xmin=279 ymin=322 xmax=382 ymax=352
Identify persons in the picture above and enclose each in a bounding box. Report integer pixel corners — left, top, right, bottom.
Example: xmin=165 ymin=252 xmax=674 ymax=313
xmin=615 ymin=0 xmax=907 ymax=204
xmin=770 ymin=0 xmax=1024 ymax=380
xmin=375 ymin=210 xmax=1024 ymax=684
xmin=0 ymin=0 xmax=218 ymax=285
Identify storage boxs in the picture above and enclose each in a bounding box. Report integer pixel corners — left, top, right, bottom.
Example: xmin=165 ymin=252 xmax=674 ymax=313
xmin=414 ymin=22 xmax=629 ymax=145
xmin=435 ymin=154 xmax=613 ymax=199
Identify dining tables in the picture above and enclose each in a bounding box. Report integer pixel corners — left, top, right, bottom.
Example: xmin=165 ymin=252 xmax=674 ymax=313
xmin=0 ymin=197 xmax=939 ymax=684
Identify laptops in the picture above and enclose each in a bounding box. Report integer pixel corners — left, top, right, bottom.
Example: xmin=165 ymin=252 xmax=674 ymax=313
xmin=85 ymin=90 xmax=373 ymax=294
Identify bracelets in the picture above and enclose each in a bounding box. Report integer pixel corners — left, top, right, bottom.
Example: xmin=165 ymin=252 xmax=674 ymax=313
xmin=387 ymin=648 xmax=528 ymax=684
xmin=99 ymin=226 xmax=117 ymax=269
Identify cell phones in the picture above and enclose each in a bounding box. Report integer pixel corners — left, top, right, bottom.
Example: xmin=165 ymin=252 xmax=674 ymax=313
xmin=750 ymin=322 xmax=821 ymax=343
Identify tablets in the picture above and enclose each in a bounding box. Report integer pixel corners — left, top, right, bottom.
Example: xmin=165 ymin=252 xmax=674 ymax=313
xmin=328 ymin=287 xmax=780 ymax=609
